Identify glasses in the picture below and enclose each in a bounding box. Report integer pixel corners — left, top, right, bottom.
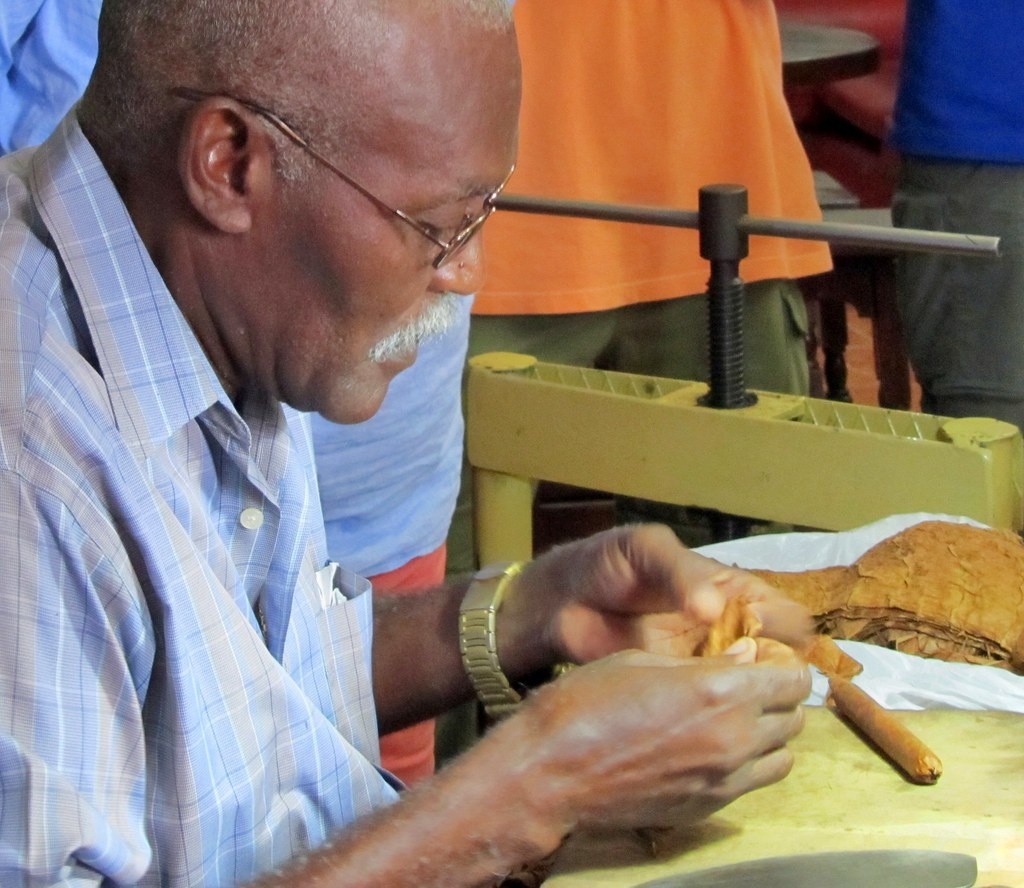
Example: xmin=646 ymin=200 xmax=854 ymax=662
xmin=170 ymin=85 xmax=517 ymax=271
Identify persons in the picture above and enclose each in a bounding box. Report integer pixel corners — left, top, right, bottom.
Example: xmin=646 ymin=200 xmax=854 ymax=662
xmin=0 ymin=1 xmax=816 ymax=888
xmin=433 ymin=1 xmax=837 ymax=776
xmin=891 ymin=0 xmax=1024 ymax=430
xmin=312 ymin=292 xmax=474 ymax=790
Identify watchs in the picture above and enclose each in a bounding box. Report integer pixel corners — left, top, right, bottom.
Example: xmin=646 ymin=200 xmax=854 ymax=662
xmin=455 ymin=559 xmax=574 ymax=722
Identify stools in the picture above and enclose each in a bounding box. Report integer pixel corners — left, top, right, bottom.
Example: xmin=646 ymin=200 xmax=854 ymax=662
xmin=811 ymin=170 xmax=912 ymax=413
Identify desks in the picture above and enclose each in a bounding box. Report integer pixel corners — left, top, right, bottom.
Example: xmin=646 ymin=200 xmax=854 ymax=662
xmin=771 ymin=19 xmax=885 ymax=85
xmin=539 ymin=703 xmax=1024 ymax=887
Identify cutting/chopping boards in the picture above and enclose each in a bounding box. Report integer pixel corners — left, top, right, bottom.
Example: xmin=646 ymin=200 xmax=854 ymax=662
xmin=538 ymin=702 xmax=1024 ymax=888
